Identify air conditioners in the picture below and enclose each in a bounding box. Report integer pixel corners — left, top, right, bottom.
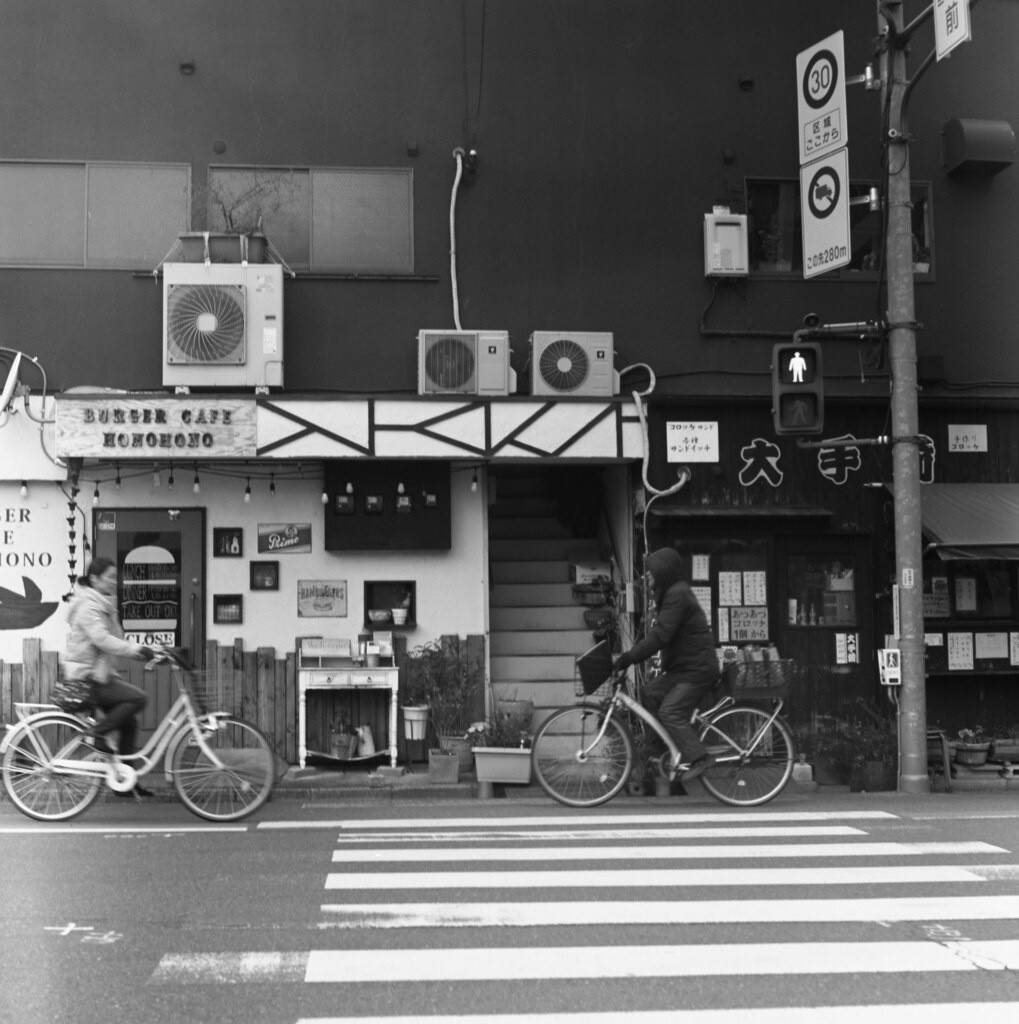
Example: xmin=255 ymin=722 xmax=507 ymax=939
xmin=529 ymin=331 xmax=614 ymax=398
xmin=417 ymin=329 xmax=511 ymax=396
xmin=159 ymin=261 xmax=285 ymax=392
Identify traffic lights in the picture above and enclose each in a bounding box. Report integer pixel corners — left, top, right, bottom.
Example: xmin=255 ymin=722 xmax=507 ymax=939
xmin=772 ymin=342 xmax=827 ymax=437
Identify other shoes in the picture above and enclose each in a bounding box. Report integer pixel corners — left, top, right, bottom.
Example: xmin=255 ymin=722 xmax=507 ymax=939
xmin=112 ymin=781 xmax=155 ymax=798
xmin=79 ymin=735 xmax=114 ymax=755
xmin=640 ymin=745 xmax=668 ymax=760
xmin=681 ymin=754 xmax=716 ymax=782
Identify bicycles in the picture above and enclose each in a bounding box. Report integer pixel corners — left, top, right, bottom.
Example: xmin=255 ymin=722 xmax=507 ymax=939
xmin=531 ymin=657 xmax=800 ymax=808
xmin=0 ymin=636 xmax=277 ymax=824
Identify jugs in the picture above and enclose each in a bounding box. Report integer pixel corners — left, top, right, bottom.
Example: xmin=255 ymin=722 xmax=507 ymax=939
xmin=355 ymin=725 xmax=376 ymax=757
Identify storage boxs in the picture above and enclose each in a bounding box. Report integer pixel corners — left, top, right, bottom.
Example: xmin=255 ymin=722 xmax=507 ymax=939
xmin=568 ymin=561 xmax=612 ymax=584
xmin=723 ymin=658 xmax=797 ymax=700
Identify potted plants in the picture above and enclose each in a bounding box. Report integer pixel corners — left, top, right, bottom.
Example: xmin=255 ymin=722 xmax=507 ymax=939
xmin=399 ymin=638 xmax=489 ymax=783
xmin=988 ymin=723 xmax=1019 ymax=763
xmin=391 ymin=592 xmax=411 ymax=626
xmin=812 ymin=698 xmax=897 ymax=794
xmin=176 ymin=162 xmax=300 ymax=264
xmin=328 ymin=709 xmax=359 ymax=758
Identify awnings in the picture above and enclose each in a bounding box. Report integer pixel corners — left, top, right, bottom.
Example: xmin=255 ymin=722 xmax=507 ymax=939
xmin=883 ymin=482 xmax=1019 ymax=560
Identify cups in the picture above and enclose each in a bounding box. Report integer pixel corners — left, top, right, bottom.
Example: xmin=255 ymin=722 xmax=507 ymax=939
xmin=366 ymin=653 xmax=380 ymax=668
xmin=391 ymin=608 xmax=407 ymax=625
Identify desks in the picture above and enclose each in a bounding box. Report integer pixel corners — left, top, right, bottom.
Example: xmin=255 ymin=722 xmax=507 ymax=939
xmin=298 ymin=666 xmax=402 ymax=769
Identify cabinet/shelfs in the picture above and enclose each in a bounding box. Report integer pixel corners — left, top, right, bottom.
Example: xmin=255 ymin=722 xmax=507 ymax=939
xmin=822 ymin=590 xmax=856 ymax=624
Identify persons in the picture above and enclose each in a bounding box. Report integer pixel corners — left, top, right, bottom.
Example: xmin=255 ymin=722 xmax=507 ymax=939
xmin=65 ymin=557 xmax=155 ymax=796
xmin=613 ymin=548 xmax=720 ymax=782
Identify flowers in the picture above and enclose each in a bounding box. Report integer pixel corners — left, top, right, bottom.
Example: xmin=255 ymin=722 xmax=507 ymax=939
xmin=463 ymin=709 xmax=532 ymax=749
xmin=958 ymin=724 xmax=987 ymax=743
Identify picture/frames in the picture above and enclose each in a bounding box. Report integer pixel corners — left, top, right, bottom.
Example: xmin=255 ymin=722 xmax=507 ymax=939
xmin=213 ymin=594 xmax=243 ymax=623
xmin=213 ymin=527 xmax=242 ymax=557
xmin=249 ymin=560 xmax=278 ymax=590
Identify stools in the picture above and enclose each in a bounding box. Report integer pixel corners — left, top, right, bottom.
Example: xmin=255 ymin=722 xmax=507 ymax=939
xmin=927 ymin=729 xmax=954 ymax=792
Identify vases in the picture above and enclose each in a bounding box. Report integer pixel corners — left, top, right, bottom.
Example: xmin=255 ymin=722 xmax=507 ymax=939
xmin=955 ymin=739 xmax=991 ymax=765
xmin=471 ymin=746 xmax=532 ymax=786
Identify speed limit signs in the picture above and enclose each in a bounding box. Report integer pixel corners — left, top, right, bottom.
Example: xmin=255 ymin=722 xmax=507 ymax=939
xmin=803 ymin=49 xmax=838 ymax=109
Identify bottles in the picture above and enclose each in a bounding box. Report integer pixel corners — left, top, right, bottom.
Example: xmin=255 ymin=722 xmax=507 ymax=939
xmin=796 ymin=602 xmax=816 ymax=626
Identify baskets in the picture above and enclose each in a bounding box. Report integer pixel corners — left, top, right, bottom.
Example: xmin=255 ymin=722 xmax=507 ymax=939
xmin=573 ymin=653 xmax=614 ymax=697
